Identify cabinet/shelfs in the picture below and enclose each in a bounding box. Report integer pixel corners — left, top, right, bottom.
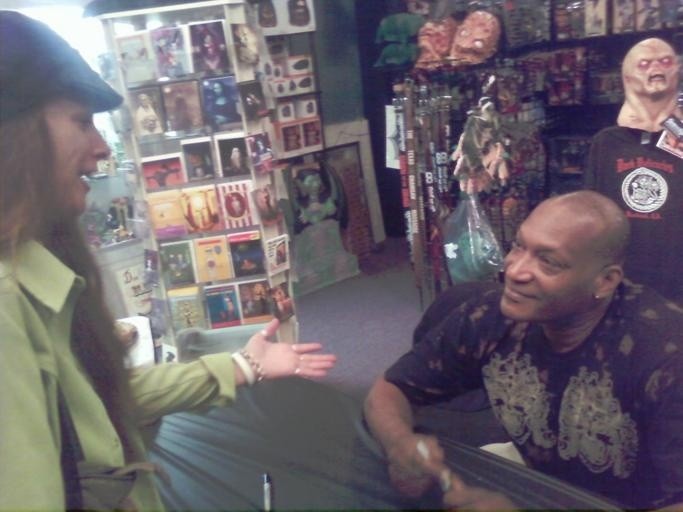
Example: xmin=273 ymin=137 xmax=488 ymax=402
xmin=361 ymin=22 xmax=682 ymax=236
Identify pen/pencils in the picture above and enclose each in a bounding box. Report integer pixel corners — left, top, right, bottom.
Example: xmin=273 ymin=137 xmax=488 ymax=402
xmin=416 ymin=439 xmax=452 ymax=492
xmin=263 ymin=474 xmax=273 ymax=512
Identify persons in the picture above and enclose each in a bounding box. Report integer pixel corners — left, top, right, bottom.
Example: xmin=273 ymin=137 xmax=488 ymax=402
xmin=0 ymin=11 xmax=338 ymax=512
xmin=363 ymin=188 xmax=682 ymax=512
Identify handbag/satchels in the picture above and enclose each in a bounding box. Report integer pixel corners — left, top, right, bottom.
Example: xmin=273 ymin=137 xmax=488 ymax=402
xmin=71 ymin=459 xmax=159 ymax=512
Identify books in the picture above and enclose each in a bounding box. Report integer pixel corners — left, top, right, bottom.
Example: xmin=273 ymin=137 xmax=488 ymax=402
xmin=116 ymin=21 xmax=294 ymax=340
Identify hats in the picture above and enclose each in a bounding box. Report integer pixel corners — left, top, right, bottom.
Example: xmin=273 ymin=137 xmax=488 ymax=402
xmin=0 ymin=9 xmax=125 ymax=121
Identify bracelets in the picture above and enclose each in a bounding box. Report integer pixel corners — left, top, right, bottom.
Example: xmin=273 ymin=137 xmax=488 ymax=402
xmin=241 ymin=350 xmax=265 ymax=382
xmin=231 ymin=351 xmax=256 ymax=385
